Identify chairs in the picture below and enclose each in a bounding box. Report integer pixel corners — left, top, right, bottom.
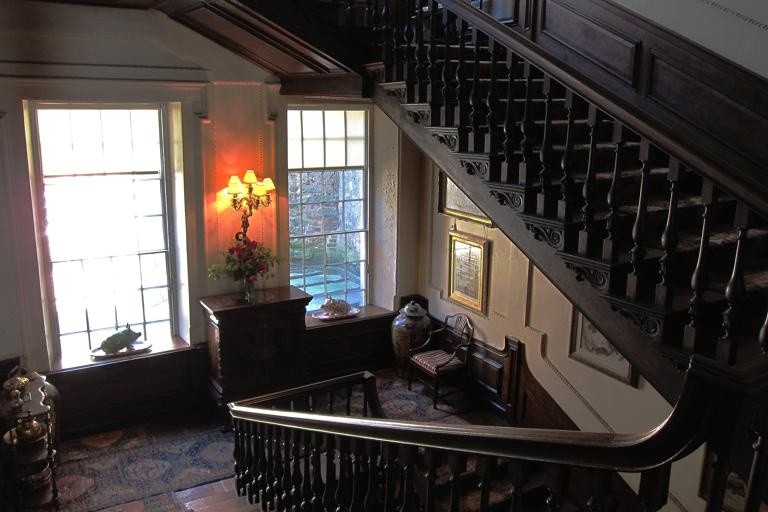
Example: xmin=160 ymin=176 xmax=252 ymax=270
xmin=405 ymin=312 xmax=475 ymax=411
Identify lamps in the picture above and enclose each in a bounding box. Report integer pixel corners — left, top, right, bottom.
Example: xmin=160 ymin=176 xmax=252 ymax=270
xmin=223 ymin=169 xmax=276 ymax=239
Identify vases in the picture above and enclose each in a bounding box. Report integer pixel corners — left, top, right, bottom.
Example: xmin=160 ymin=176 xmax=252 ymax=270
xmin=241 ymin=278 xmax=257 ymax=304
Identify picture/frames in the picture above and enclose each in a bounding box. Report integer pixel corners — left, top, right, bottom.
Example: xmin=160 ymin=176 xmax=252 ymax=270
xmin=436 ymin=169 xmax=493 ymax=316
xmin=567 ymin=301 xmax=639 ymax=389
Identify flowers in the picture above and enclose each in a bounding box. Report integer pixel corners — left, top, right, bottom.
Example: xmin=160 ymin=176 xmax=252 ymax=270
xmin=198 ymin=241 xmax=287 ymax=287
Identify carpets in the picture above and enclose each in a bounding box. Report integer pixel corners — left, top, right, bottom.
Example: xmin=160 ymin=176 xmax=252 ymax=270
xmin=44 ymin=367 xmax=466 ymax=511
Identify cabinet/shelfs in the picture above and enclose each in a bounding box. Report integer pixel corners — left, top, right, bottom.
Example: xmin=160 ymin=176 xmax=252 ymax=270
xmin=198 ymin=289 xmax=313 ymax=433
